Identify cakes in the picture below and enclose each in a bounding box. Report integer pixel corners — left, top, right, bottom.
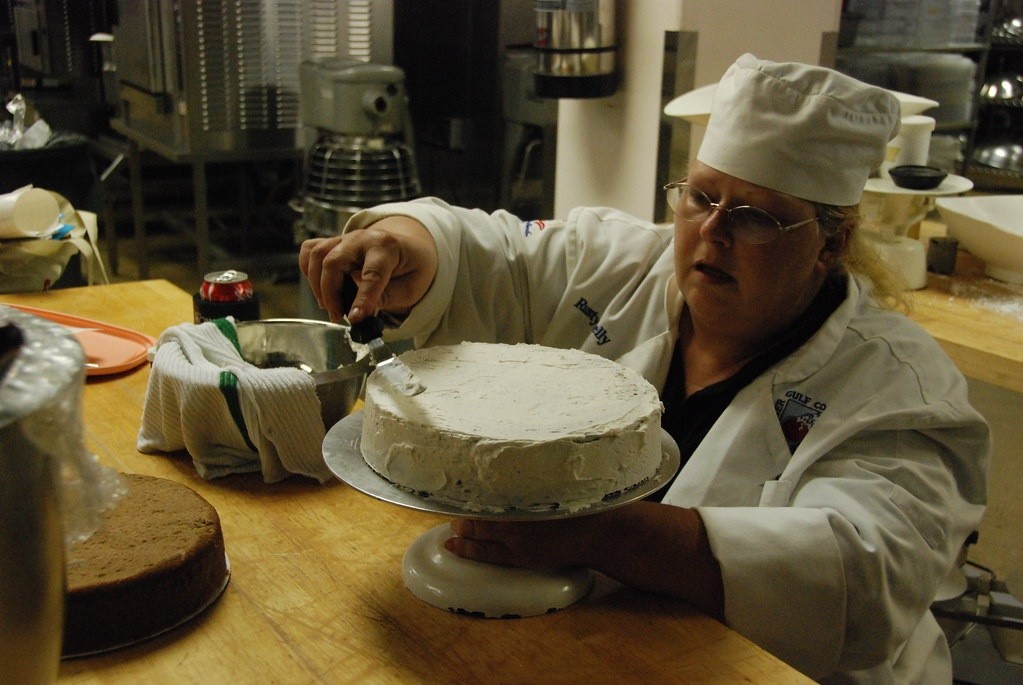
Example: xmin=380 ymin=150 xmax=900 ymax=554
xmin=60 ymin=474 xmax=225 ymax=656
xmin=360 ymin=338 xmax=667 ymax=508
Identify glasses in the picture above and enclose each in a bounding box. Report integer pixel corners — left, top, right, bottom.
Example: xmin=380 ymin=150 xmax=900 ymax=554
xmin=663 ymin=177 xmax=830 ymax=245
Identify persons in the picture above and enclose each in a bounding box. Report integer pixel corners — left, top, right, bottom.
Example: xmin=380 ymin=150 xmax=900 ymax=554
xmin=298 ymin=51 xmax=992 ymax=685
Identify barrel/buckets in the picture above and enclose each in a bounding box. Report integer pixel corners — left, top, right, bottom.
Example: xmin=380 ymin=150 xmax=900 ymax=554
xmin=880 ymin=115 xmax=937 ymax=178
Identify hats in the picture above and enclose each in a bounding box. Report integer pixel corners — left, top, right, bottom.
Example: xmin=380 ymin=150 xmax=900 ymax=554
xmin=697 ymin=53 xmax=901 ymax=207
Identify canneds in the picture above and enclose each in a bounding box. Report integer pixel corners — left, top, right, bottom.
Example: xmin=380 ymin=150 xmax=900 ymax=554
xmin=200 ymin=270 xmax=254 ymax=303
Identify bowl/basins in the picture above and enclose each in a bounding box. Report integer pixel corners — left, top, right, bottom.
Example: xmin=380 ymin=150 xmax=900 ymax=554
xmin=934 ymin=194 xmax=1023 ymax=282
xmin=970 ymin=139 xmax=1023 ymax=172
xmin=978 ymin=77 xmax=1023 ymax=101
xmin=154 ymin=317 xmax=376 ymax=430
xmin=889 ymin=165 xmax=948 ymax=189
xmin=992 ymin=14 xmax=1023 ymax=41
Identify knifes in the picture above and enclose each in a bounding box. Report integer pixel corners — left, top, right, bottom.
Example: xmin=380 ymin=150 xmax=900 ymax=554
xmin=337 ymin=274 xmax=426 ymax=398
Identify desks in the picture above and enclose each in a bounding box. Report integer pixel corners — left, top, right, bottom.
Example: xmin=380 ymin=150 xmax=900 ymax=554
xmin=0 ymin=276 xmax=825 ymax=683
